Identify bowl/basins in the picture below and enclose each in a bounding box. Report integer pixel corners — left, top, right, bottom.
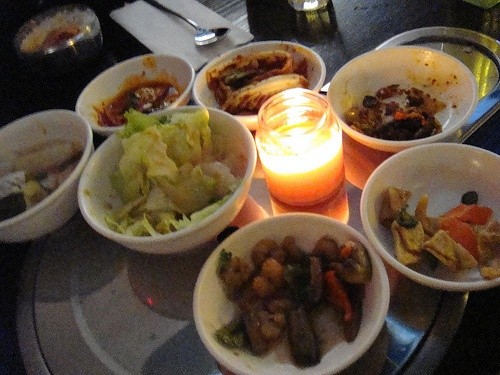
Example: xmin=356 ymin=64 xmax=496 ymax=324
xmin=78 ymin=105 xmax=258 ymax=254
xmin=0 ymin=108 xmax=95 ymax=243
xmin=192 ymin=211 xmax=390 ymax=374
xmin=191 ymin=40 xmax=326 ymax=131
xmin=328 ymin=44 xmax=478 ymax=152
xmin=74 ymin=52 xmax=195 ymax=136
xmin=360 ymin=141 xmax=500 ymax=291
xmin=12 ymin=3 xmax=102 ymax=65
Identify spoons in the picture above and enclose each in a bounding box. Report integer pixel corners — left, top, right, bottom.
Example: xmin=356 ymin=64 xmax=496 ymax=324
xmin=143 ymin=0 xmax=231 ymax=46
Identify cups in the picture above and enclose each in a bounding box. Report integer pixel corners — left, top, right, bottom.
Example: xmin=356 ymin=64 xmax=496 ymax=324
xmin=254 ymin=86 xmax=345 ymax=206
xmin=288 ymin=0 xmax=333 ymax=12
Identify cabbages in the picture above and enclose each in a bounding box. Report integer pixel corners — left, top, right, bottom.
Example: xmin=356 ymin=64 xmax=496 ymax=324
xmin=104 ymin=105 xmax=241 ymax=240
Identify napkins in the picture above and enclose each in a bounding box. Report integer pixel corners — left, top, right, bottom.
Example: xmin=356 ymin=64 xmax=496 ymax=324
xmin=109 ymin=0 xmax=255 ymax=72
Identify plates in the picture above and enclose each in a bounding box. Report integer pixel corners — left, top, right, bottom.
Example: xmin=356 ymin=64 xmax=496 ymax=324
xmin=373 ymin=27 xmax=500 ymax=98
xmin=109 ymin=0 xmax=254 ymax=73
